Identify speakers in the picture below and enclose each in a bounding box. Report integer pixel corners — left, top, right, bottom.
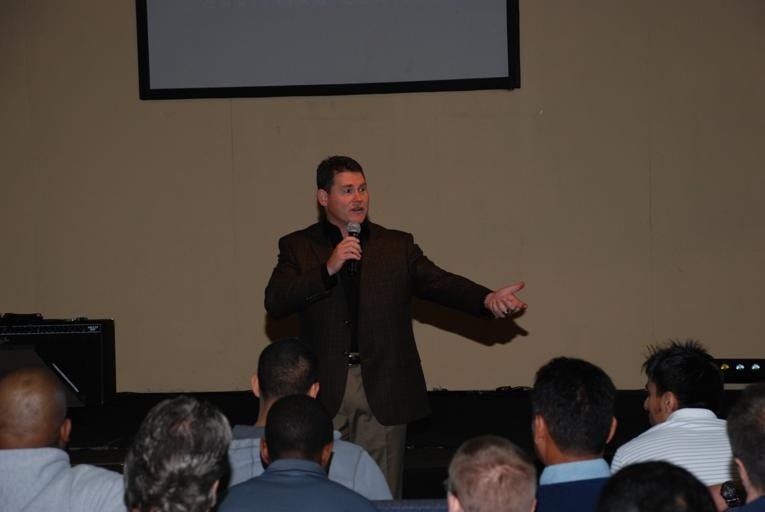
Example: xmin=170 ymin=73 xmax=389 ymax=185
xmin=0 ymin=313 xmax=115 ymax=409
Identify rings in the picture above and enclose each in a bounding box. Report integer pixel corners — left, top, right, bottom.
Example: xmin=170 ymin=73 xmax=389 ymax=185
xmin=503 ymin=308 xmax=510 ymax=314
xmin=512 ymin=305 xmax=518 ymax=313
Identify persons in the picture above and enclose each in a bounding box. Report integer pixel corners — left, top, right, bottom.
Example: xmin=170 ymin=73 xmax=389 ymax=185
xmin=123 ymin=396 xmax=235 ymax=512
xmin=264 ymin=156 xmax=528 ymax=499
xmin=1 ymin=368 xmax=127 ymax=510
xmin=531 ymin=341 xmax=765 ymax=512
xmin=447 ymin=437 xmax=537 ymax=511
xmin=215 ymin=395 xmax=375 ymax=510
xmin=225 ymin=337 xmax=392 ymax=503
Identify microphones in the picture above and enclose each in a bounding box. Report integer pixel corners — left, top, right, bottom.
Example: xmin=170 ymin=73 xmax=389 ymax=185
xmin=347 ymin=221 xmax=361 ymax=277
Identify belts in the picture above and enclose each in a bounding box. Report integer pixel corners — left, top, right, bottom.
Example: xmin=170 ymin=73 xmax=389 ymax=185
xmin=347 ymin=353 xmax=360 ymax=368
